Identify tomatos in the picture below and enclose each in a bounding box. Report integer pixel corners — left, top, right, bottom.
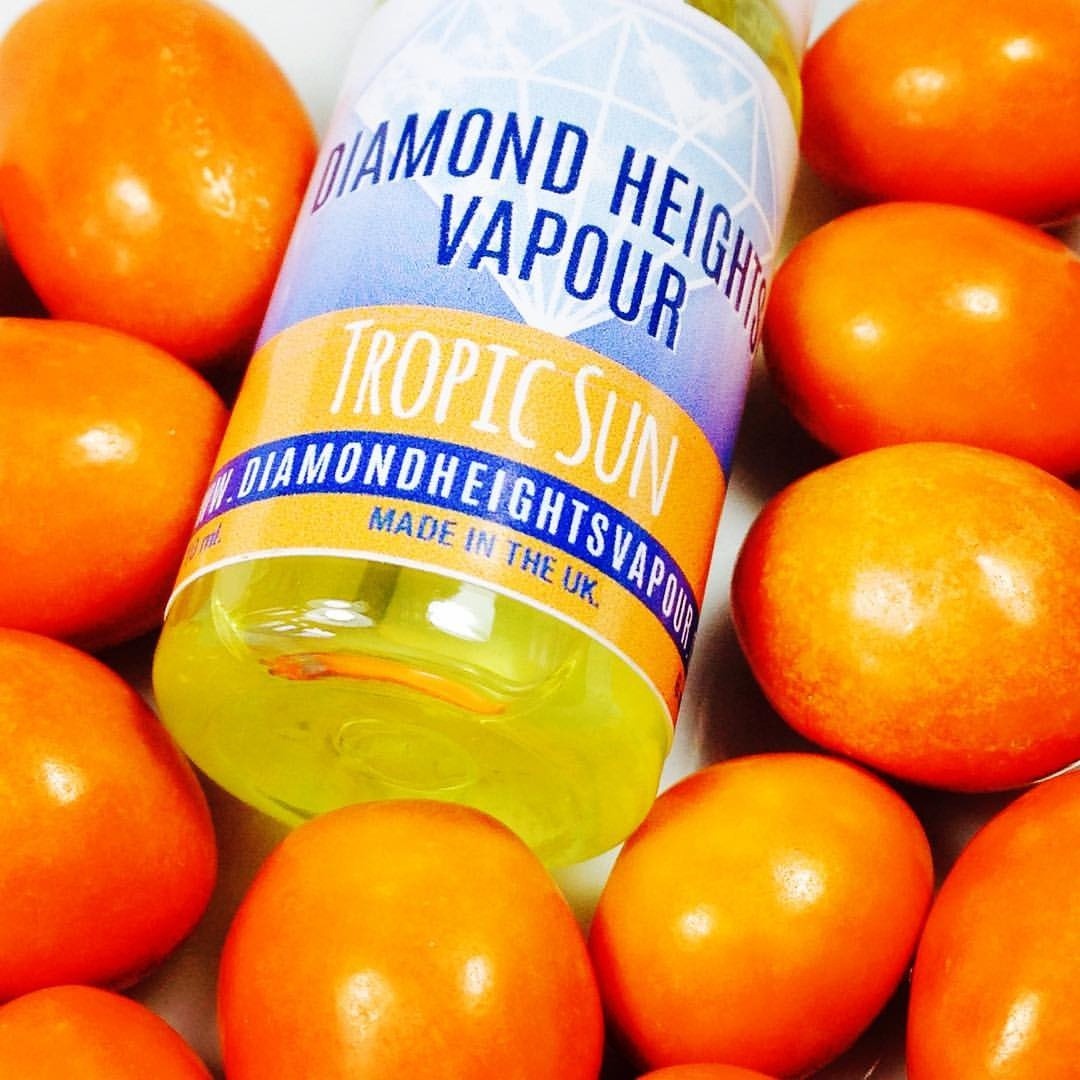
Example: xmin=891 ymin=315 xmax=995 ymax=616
xmin=0 ymin=0 xmax=1080 ymax=1080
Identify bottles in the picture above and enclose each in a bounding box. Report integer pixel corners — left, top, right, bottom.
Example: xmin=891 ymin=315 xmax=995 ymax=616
xmin=151 ymin=1 xmax=818 ymax=879
